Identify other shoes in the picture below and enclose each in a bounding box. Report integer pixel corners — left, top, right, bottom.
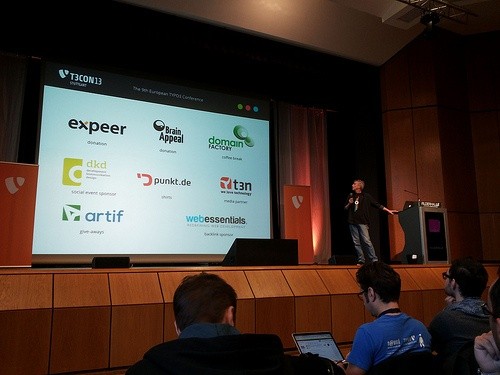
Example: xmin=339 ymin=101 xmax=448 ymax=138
xmin=357 ymin=262 xmax=365 ymax=266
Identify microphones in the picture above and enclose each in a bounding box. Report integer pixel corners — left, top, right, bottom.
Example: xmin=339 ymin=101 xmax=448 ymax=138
xmin=405 ymin=190 xmax=420 ymax=201
xmin=350 ymin=193 xmax=353 ymax=198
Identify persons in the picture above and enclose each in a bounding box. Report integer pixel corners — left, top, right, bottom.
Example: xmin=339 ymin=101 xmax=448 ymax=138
xmin=472 ymin=278 xmax=500 ymax=375
xmin=124 ymin=270 xmax=346 ymax=375
xmin=342 ymin=178 xmax=399 ymax=268
xmin=428 ymin=257 xmax=491 ymax=375
xmin=334 ymin=261 xmax=435 ymax=375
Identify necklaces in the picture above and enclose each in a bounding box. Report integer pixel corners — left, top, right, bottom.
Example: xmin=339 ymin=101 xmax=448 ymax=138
xmin=377 ymin=308 xmax=402 ymax=318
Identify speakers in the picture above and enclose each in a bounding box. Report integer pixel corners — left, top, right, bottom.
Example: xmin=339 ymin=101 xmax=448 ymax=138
xmin=223 ymin=237 xmax=298 ymax=266
xmin=91 ymin=257 xmax=131 ymax=269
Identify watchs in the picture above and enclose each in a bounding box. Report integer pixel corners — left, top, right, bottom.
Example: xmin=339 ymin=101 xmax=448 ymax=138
xmin=477 ymin=368 xmax=497 ymax=374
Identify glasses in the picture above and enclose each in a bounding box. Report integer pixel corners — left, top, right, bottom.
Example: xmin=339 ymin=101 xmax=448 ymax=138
xmin=357 ymin=289 xmax=365 ymax=302
xmin=442 ymin=272 xmax=452 ymax=281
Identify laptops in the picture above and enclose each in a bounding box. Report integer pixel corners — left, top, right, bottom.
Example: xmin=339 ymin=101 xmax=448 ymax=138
xmin=291 ymin=331 xmax=345 ymax=364
